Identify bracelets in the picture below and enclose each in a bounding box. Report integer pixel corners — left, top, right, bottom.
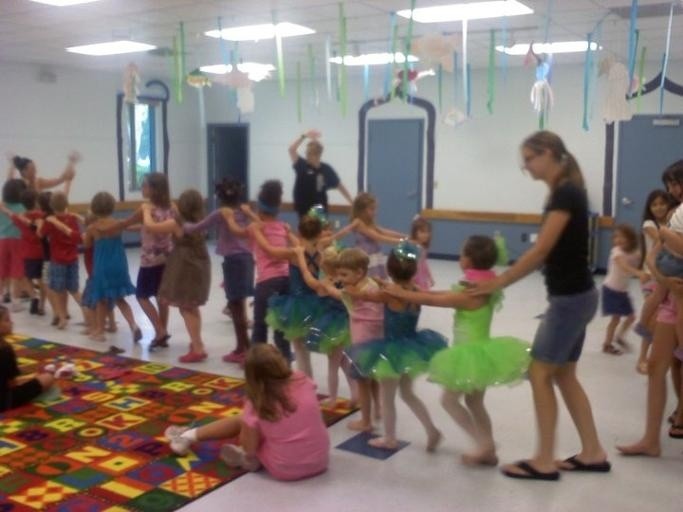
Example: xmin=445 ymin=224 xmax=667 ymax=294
xmin=504 ymin=270 xmax=516 ymax=283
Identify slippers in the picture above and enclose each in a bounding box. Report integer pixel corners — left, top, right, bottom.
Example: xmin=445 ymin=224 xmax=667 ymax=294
xmin=225 ymin=351 xmax=244 ymax=365
xmin=668 ymin=414 xmax=682 ymax=441
xmin=149 ymin=332 xmax=169 ymax=350
xmin=504 ymin=462 xmax=559 ymax=481
xmin=181 ymin=353 xmax=206 ymax=363
xmin=562 ymin=457 xmax=613 ymax=473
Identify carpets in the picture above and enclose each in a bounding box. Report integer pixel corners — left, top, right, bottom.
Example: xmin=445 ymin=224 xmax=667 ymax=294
xmin=0 ymin=335 xmax=366 ymax=512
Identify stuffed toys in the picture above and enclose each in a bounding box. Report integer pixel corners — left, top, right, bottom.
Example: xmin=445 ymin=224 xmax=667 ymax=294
xmin=183 ymin=67 xmax=212 ymax=90
xmin=526 ymin=39 xmax=553 ymax=130
xmin=597 ymin=57 xmax=646 ymax=126
xmin=122 ymin=62 xmax=142 ymax=104
xmin=210 ymin=42 xmax=251 ymax=104
xmin=391 ymin=67 xmax=435 ymax=93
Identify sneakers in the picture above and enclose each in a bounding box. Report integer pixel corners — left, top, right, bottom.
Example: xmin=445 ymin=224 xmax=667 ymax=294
xmin=165 ymin=424 xmax=192 ymax=459
xmin=220 ymin=444 xmax=262 ymax=474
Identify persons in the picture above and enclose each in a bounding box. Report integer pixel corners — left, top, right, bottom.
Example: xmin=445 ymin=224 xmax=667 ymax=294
xmin=247 ymin=214 xmax=324 ymax=380
xmin=165 ymin=340 xmax=331 ymax=482
xmin=463 ymin=130 xmax=612 ymax=481
xmin=351 ymin=192 xmax=409 ymax=282
xmin=0 ymin=189 xmax=45 ymax=315
xmin=0 ymin=178 xmax=29 ymax=312
xmin=142 ymin=189 xmax=211 ymax=364
xmin=16 ymin=191 xmax=71 ymax=326
xmin=319 ymin=217 xmax=361 ymax=281
xmin=636 ymin=189 xmax=674 ymax=376
xmin=94 ymin=171 xmax=173 ymax=352
xmin=175 ymin=173 xmax=255 ymax=364
xmin=294 ymin=244 xmax=361 ymax=411
xmin=342 ymin=236 xmax=448 ymax=454
xmin=0 ymin=305 xmax=54 ymax=413
xmin=616 ymin=160 xmax=683 ymax=458
xmin=321 ymin=247 xmax=384 ymax=432
xmin=634 ymin=200 xmax=683 ymax=363
xmin=600 ymin=223 xmax=651 ymax=356
xmin=34 ymin=192 xmax=92 ymax=330
xmin=288 ymin=130 xmax=354 ymax=220
xmin=222 ymin=180 xmax=295 ymax=367
xmin=383 ymin=234 xmax=533 ymax=467
xmin=411 ymin=213 xmax=435 ymax=290
xmin=46 ymin=212 xmax=117 ymax=335
xmin=9 ymin=156 xmax=74 ymax=194
xmin=82 ymin=191 xmax=143 ymax=343
